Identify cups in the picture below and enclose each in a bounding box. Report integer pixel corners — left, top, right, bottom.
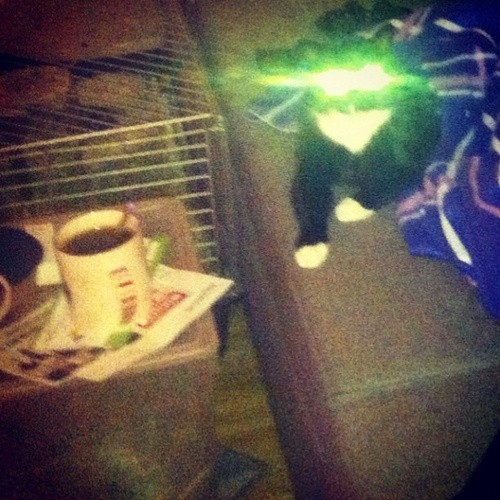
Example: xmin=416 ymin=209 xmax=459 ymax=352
xmin=52 ymin=209 xmax=150 ymax=331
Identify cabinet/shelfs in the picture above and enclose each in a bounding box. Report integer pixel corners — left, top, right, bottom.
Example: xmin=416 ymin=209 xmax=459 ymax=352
xmin=0 ymin=194 xmax=226 ymax=495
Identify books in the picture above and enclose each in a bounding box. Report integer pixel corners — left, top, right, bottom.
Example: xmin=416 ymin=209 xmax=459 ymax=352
xmin=1 ymin=195 xmax=238 ymax=402
